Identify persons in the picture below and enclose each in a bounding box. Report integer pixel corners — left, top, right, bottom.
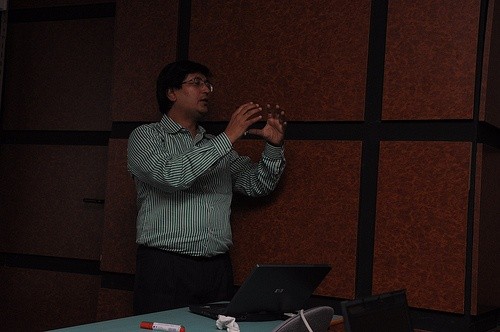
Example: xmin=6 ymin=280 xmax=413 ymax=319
xmin=128 ymin=60 xmax=287 ymax=314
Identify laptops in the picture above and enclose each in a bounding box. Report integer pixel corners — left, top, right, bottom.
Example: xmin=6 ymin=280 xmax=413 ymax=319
xmin=188 ymin=264 xmax=332 ymax=322
xmin=341 ymin=288 xmax=414 ymax=332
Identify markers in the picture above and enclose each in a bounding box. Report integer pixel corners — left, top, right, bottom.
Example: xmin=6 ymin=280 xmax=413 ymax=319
xmin=139 ymin=321 xmax=185 ymax=332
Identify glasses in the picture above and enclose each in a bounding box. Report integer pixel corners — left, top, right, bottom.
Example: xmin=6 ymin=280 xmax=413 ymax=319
xmin=182 ymin=76 xmax=213 ymax=93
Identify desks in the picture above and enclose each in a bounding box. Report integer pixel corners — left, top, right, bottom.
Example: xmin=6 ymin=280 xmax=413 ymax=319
xmin=44 ymin=301 xmax=345 ymax=332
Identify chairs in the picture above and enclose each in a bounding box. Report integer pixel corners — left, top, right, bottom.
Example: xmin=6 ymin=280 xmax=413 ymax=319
xmin=274 ymin=306 xmax=334 ymax=332
